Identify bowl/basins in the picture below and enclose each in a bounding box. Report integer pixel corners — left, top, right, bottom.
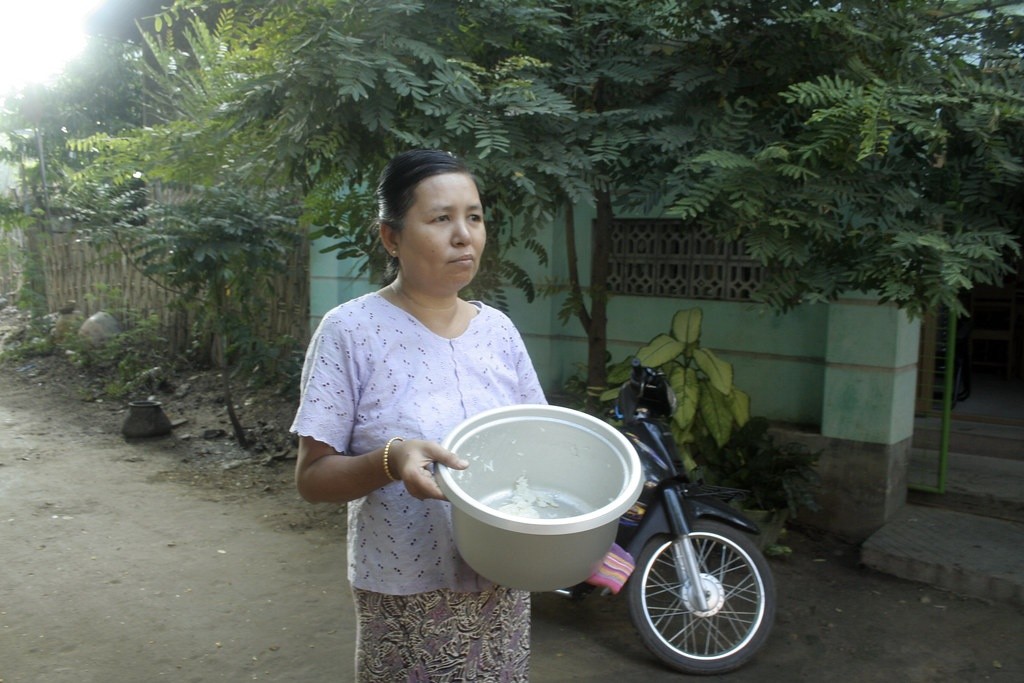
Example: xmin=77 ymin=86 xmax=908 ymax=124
xmin=434 ymin=403 xmax=645 ymax=592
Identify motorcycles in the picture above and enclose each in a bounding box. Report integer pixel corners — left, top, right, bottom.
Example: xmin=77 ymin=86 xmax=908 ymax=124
xmin=535 ymin=356 xmax=780 ymax=675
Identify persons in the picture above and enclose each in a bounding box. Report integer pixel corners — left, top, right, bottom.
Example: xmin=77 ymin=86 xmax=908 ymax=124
xmin=289 ymin=149 xmax=549 ymax=683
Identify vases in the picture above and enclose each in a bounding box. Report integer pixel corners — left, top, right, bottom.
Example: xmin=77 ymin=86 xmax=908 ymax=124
xmin=122 ymin=401 xmax=173 ymax=440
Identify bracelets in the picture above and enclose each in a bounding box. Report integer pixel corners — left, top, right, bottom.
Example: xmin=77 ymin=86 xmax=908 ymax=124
xmin=383 ymin=435 xmax=403 ymax=482
